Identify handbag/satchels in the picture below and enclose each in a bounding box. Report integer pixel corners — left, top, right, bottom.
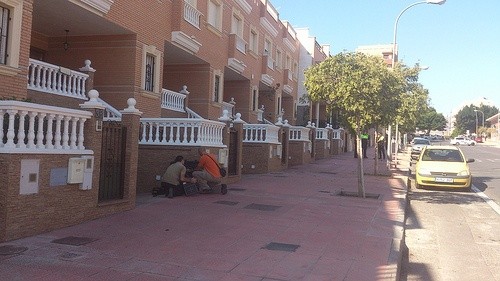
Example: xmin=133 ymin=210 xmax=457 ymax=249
xmin=220 ymin=169 xmax=226 ymax=177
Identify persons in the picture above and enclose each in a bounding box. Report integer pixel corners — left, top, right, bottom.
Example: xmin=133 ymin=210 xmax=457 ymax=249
xmin=361 ymin=130 xmax=370 ymax=158
xmin=192 ymin=146 xmax=227 ymax=195
xmin=377 ymin=132 xmax=386 ymax=159
xmin=161 ymin=155 xmax=196 ymax=199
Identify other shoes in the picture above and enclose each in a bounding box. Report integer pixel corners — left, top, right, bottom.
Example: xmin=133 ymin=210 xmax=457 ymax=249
xmin=168 ymin=187 xmax=173 ymax=197
xmin=200 ymin=189 xmax=213 ymax=194
xmin=221 ymin=184 xmax=227 ymax=195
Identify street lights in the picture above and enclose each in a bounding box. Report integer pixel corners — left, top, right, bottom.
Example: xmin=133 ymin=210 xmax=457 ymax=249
xmin=473 ymin=110 xmax=484 ymax=127
xmin=386 ymin=0 xmax=448 ymax=166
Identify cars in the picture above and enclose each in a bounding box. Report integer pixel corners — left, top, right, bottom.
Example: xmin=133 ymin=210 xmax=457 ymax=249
xmin=410 ymin=136 xmax=431 ymax=154
xmin=449 ymin=134 xmax=485 ymax=146
xmin=415 ymin=145 xmax=476 ymax=191
